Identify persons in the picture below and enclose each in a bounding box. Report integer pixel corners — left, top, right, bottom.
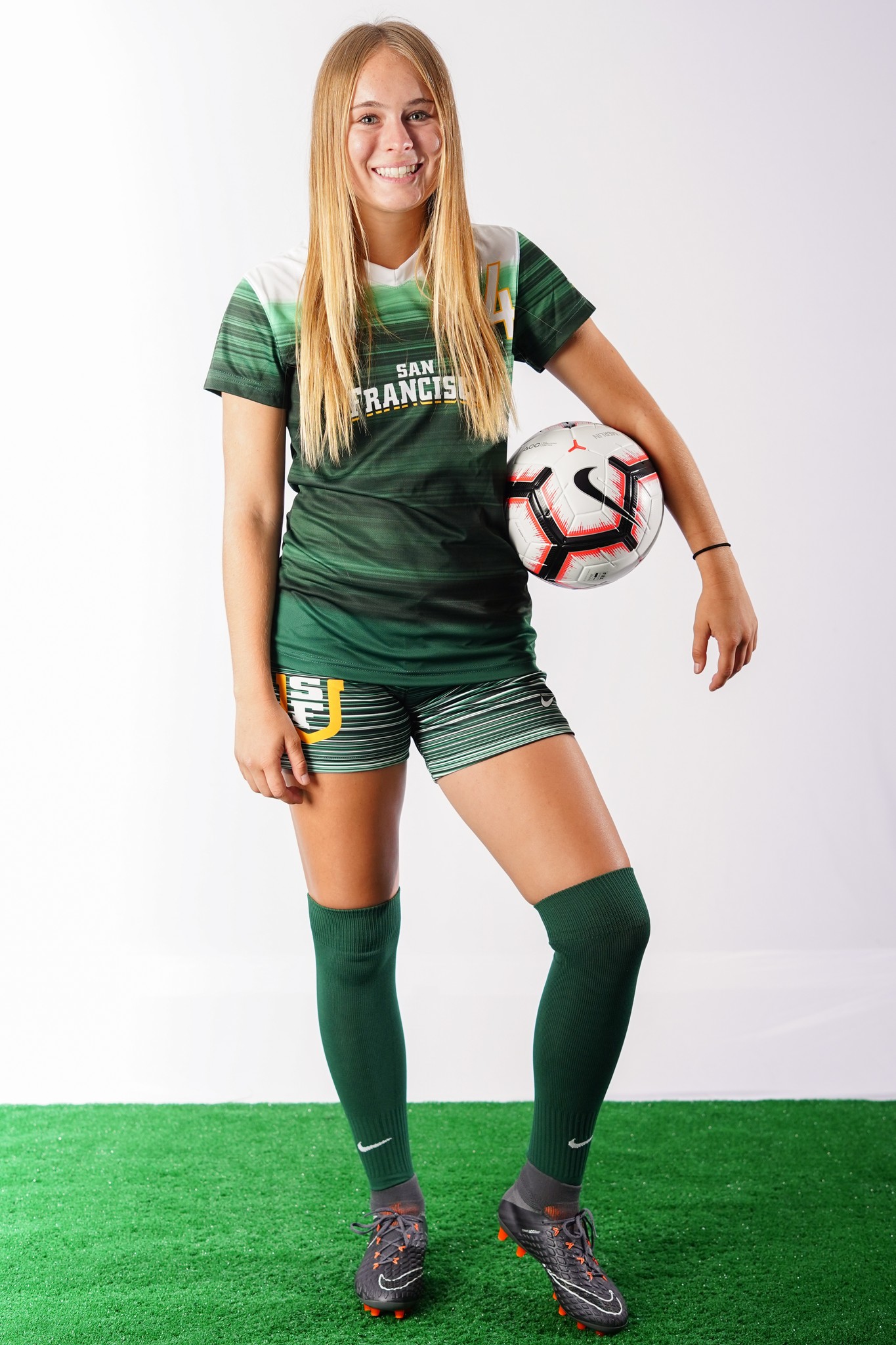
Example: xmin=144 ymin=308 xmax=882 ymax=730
xmin=204 ymin=20 xmax=759 ymax=1337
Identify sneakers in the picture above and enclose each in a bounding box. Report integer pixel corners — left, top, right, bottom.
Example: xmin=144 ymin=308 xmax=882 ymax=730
xmin=496 ymin=1157 xmax=629 ymax=1336
xmin=351 ymin=1172 xmax=428 ymax=1318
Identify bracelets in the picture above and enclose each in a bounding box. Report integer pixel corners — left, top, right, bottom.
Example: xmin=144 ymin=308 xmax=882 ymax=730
xmin=692 ymin=542 xmax=731 ymax=559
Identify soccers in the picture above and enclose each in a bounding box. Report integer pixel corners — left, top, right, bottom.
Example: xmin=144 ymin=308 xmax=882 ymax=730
xmin=505 ymin=420 xmax=666 ymax=590
